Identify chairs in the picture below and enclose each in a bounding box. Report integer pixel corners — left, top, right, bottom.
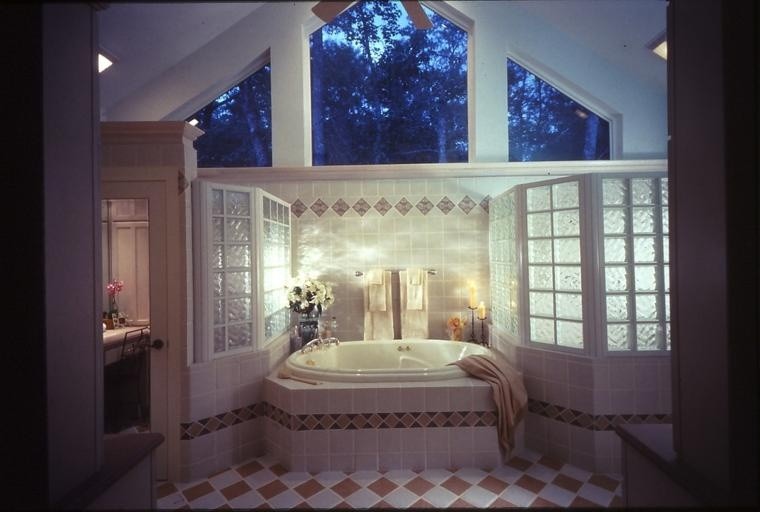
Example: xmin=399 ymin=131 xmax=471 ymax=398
xmin=104 ymin=324 xmax=150 ymax=433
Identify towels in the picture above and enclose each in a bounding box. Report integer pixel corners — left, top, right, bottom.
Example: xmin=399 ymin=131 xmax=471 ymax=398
xmin=362 ymin=269 xmax=395 ymax=341
xmin=445 ymin=353 xmax=530 ymax=461
xmin=399 ymin=267 xmax=429 ymax=339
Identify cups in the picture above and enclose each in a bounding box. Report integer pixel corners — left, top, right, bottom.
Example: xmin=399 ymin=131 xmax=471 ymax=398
xmin=112 ymin=312 xmax=128 ymax=330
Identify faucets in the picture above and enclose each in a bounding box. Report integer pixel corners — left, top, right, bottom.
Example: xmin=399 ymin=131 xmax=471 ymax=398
xmin=301 ymin=337 xmax=323 ymax=352
xmin=322 ymin=337 xmax=339 ymax=346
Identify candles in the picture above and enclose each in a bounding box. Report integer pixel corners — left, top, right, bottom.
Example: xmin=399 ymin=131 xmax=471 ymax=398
xmin=469 ymin=286 xmax=477 ymax=308
xmin=478 ymin=303 xmax=485 ymax=319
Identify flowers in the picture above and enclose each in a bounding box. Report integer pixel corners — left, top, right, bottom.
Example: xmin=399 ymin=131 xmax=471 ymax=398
xmin=287 ymin=278 xmax=335 ymax=319
xmin=447 ymin=316 xmax=469 ymax=336
xmin=105 ymin=279 xmax=124 ymax=298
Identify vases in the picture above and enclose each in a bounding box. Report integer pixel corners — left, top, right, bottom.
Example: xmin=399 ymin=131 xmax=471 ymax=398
xmin=452 ymin=328 xmax=464 ymax=341
xmin=299 ymin=311 xmax=320 ymax=348
xmin=111 ymin=296 xmax=118 ymax=315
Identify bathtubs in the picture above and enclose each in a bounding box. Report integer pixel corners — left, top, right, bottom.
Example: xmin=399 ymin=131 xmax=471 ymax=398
xmin=284 ymin=339 xmax=495 ymax=382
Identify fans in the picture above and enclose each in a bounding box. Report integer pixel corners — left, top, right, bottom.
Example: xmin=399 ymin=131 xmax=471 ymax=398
xmin=311 ymin=0 xmax=433 ymax=30
